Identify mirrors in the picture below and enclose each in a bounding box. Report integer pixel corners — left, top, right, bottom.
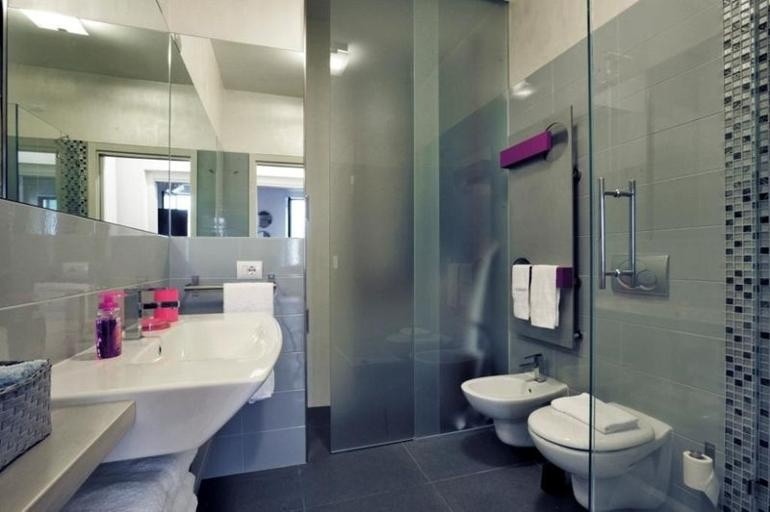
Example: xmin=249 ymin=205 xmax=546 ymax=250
xmin=1 ymin=7 xmax=305 ymax=237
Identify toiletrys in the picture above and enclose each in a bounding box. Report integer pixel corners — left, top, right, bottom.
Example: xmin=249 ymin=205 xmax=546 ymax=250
xmin=94 ymin=291 xmax=131 ymax=359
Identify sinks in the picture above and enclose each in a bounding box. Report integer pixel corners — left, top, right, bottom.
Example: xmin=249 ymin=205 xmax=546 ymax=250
xmin=49 ymin=311 xmax=284 ymax=465
xmin=460 ymin=371 xmax=569 ymax=448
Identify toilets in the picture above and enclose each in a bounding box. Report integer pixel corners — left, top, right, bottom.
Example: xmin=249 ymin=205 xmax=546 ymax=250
xmin=527 ymin=401 xmax=673 ymax=512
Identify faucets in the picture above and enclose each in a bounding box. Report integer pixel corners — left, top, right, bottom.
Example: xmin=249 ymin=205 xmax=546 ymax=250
xmin=123 ymin=287 xmax=180 ymax=341
xmin=519 ymin=352 xmax=544 ymax=380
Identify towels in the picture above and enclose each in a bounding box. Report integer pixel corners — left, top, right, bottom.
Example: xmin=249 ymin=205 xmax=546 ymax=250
xmin=551 ymin=391 xmax=638 ymax=434
xmin=529 ymin=264 xmax=561 ymax=329
xmin=510 ymin=264 xmax=531 ymax=321
xmin=223 ymin=281 xmax=274 ymax=405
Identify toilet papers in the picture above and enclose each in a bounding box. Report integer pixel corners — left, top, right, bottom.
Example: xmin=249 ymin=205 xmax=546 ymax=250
xmin=682 ymin=449 xmax=721 ymax=510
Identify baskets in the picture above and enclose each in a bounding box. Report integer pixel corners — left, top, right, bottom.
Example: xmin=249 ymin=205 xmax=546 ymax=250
xmin=0 ymin=358 xmax=54 ymax=475
